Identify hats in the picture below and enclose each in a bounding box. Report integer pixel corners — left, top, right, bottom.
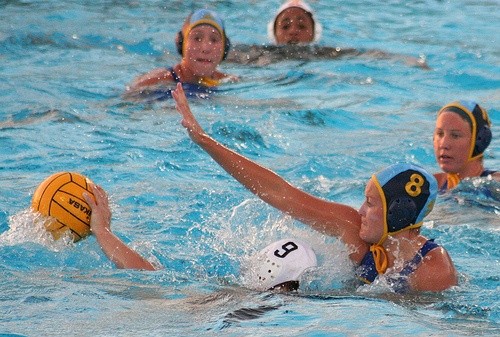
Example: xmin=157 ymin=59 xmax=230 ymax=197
xmin=371 ymin=162 xmax=439 ymax=234
xmin=176 ymin=8 xmax=231 ymax=65
xmin=437 ymin=96 xmax=493 ymax=160
xmin=257 ymin=238 xmax=318 ymax=291
xmin=268 ymin=0 xmax=322 ymax=46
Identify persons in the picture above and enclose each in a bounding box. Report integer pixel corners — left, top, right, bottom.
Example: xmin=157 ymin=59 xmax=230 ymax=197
xmin=171 ymin=80 xmax=459 ymax=295
xmin=424 ymin=99 xmax=500 ymax=212
xmin=1 ymin=7 xmax=324 ymax=130
xmin=231 ymin=0 xmax=429 ymax=71
xmin=83 ymin=182 xmax=318 ymax=331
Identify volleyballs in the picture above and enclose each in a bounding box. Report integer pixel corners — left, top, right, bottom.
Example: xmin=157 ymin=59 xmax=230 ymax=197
xmin=29 ymin=171 xmax=97 ymax=244
